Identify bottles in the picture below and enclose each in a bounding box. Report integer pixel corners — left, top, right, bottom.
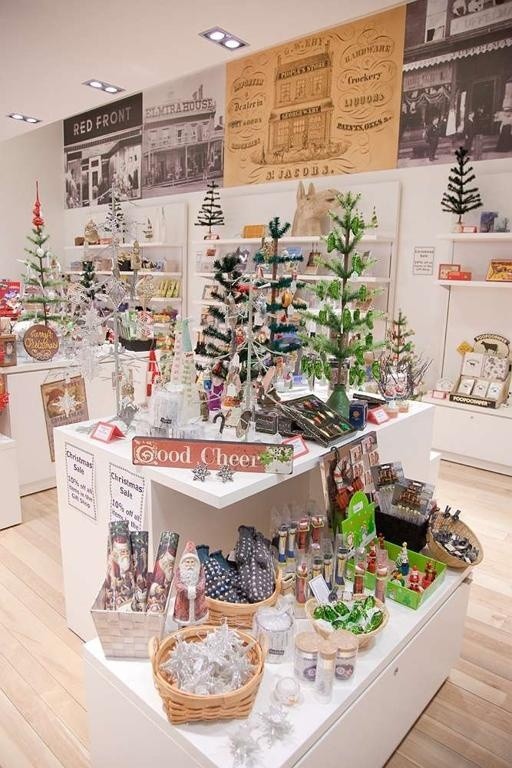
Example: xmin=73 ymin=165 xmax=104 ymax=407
xmin=251 ymin=604 xmax=358 ymax=702
xmin=159 ymin=206 xmax=169 ymax=242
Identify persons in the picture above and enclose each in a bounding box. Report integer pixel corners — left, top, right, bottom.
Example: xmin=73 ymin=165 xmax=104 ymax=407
xmin=398 ymin=101 xmax=512 ymax=162
xmin=66 ymin=168 xmax=79 ymax=209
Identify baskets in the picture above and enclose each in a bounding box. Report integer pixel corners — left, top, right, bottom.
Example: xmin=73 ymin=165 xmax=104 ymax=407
xmin=304 ymin=592 xmax=391 ymax=655
xmin=426 ymin=511 xmax=484 ymax=569
xmin=203 ymin=550 xmax=282 ymax=629
xmin=117 ymin=335 xmax=156 ymax=352
xmin=151 ymin=624 xmax=267 ymax=726
